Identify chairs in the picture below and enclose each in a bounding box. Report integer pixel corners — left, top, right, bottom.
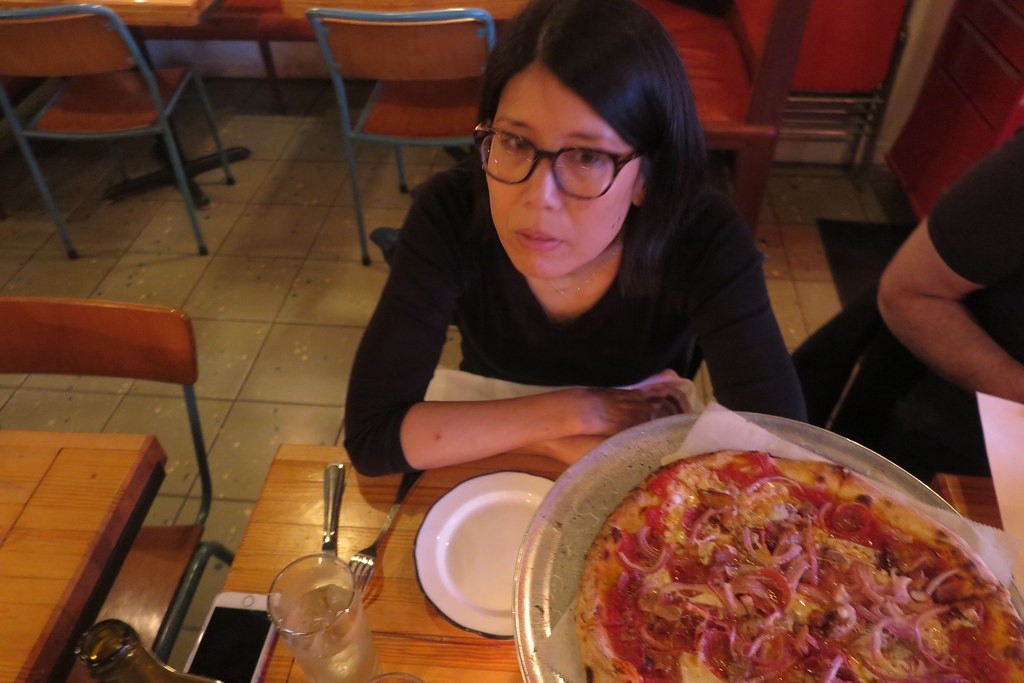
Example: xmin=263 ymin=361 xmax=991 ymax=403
xmin=0 ymin=4 xmax=236 ymax=260
xmin=305 ymin=7 xmax=497 ymax=266
xmin=733 ymin=0 xmax=915 ymax=191
xmin=639 ymin=0 xmax=812 ymax=243
xmin=0 ymin=295 xmax=234 ymax=683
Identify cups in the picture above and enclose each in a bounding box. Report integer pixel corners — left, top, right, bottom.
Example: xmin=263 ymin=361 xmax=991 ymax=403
xmin=266 ymin=553 xmax=376 ymax=683
xmin=368 ymin=672 xmax=424 ymax=683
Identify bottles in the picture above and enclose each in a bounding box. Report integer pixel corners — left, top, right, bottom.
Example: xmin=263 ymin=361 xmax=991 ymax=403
xmin=74 ymin=618 xmax=219 ymax=682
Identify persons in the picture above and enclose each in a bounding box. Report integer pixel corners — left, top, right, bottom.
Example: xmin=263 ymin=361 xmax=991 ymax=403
xmin=876 ymin=132 xmax=1024 ymax=479
xmin=341 ymin=0 xmax=807 ymax=475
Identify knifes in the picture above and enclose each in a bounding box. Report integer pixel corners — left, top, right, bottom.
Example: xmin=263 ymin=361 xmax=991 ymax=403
xmin=317 ymin=461 xmax=344 ymax=568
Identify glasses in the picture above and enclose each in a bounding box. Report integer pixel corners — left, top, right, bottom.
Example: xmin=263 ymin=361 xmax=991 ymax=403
xmin=473 ymin=116 xmax=650 ymax=201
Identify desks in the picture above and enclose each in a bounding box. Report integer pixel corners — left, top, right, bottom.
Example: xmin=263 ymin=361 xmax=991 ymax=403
xmin=219 ymin=442 xmax=570 ymax=683
xmin=0 ymin=430 xmax=169 ymax=683
xmin=0 ymin=0 xmax=556 ymax=115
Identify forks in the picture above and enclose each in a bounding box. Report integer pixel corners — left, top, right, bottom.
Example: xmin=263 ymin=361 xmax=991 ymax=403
xmin=347 ymin=468 xmax=424 ymax=597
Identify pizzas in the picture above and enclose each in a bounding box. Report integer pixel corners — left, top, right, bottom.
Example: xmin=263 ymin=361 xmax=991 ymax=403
xmin=572 ymin=449 xmax=1024 ymax=683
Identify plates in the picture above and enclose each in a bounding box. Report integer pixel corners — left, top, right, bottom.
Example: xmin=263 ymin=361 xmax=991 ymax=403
xmin=510 ymin=411 xmax=984 ymax=683
xmin=414 ymin=469 xmax=556 ymax=639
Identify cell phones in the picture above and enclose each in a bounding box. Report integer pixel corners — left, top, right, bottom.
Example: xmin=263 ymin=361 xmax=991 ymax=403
xmin=183 ymin=591 xmax=280 ymax=683
